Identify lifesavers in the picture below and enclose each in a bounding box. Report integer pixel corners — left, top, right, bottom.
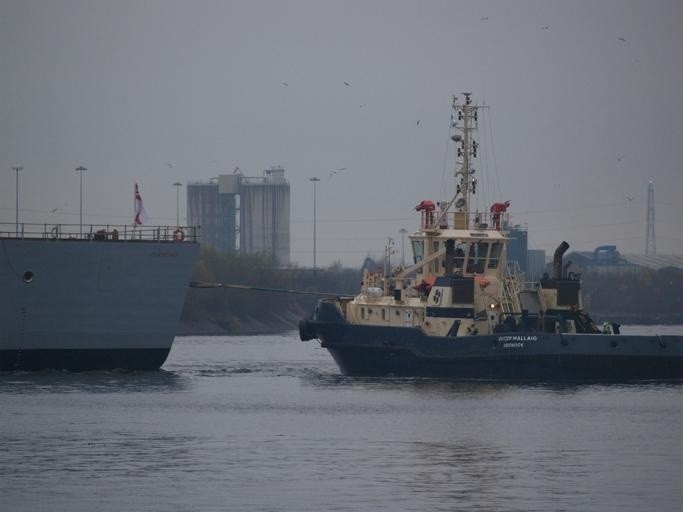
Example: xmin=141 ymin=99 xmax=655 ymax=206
xmin=173 ymin=229 xmax=184 ymax=241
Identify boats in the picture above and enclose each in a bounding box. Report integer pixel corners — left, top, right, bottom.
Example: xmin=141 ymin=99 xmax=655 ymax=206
xmin=290 ymin=86 xmax=683 ymax=381
xmin=0 ymin=221 xmax=200 ymax=379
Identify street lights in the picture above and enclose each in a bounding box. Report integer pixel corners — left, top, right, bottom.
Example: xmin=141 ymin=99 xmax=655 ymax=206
xmin=397 ymin=226 xmax=406 ymax=266
xmin=306 ymin=175 xmax=318 ymax=277
xmin=75 ymin=165 xmax=88 ymax=236
xmin=10 ymin=167 xmax=24 ymax=237
xmin=172 ymin=182 xmax=181 ymax=227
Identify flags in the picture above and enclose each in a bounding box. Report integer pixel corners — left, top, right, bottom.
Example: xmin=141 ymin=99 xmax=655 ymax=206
xmin=132 ymin=184 xmax=147 ymax=225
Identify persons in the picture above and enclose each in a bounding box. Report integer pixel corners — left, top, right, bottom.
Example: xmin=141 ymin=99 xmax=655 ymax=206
xmin=611 ymin=319 xmax=621 ymax=334
xmin=606 ymin=325 xmax=612 ymax=334
xmin=415 ymin=200 xmax=436 ymax=227
xmin=602 ymin=318 xmax=610 ymax=334
xmin=490 ymin=200 xmax=512 ymax=230
